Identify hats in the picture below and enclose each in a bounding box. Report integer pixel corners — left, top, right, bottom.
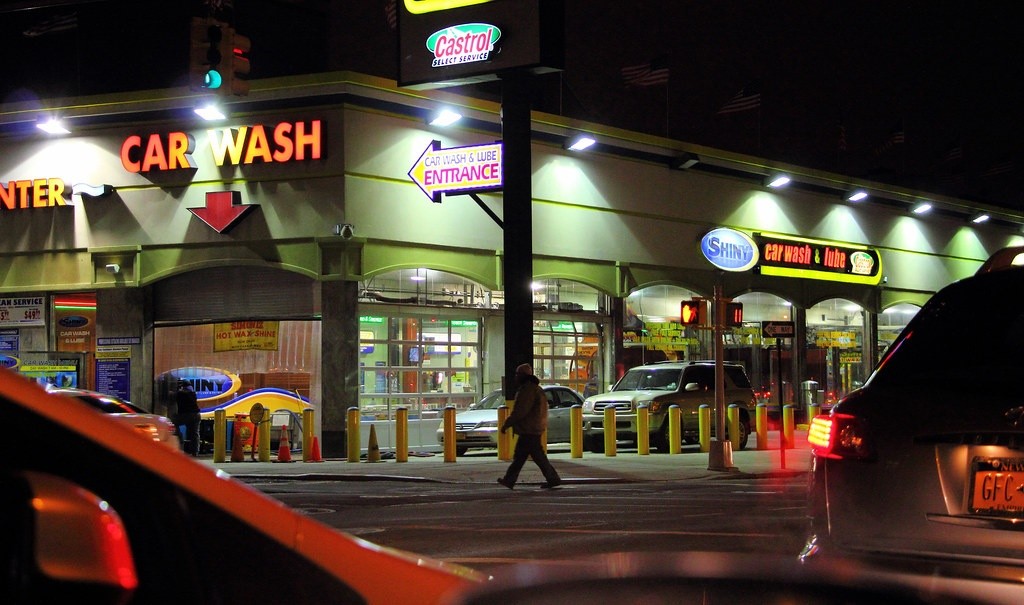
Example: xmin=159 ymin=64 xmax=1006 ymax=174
xmin=183 ymin=379 xmax=191 ymax=386
xmin=517 ymin=363 xmax=533 ymax=375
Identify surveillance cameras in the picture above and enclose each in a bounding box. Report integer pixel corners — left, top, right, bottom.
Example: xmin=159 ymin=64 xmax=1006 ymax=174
xmin=334 ymin=224 xmax=354 ymax=238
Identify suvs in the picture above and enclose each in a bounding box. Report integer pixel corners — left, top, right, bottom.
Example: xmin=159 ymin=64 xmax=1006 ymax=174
xmin=581 ymin=361 xmax=757 ymax=452
xmin=796 ymin=247 xmax=1022 ymax=604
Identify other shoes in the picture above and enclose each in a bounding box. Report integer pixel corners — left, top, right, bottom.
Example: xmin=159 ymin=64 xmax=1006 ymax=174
xmin=193 ymin=453 xmax=199 ymax=456
xmin=497 ymin=478 xmax=514 ymax=489
xmin=540 ymin=480 xmax=563 ymax=489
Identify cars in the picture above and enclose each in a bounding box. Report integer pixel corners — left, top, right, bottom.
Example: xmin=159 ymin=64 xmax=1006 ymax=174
xmin=0 ymin=365 xmax=503 ymax=604
xmin=52 ymin=387 xmax=181 ymax=450
xmin=436 ymin=384 xmax=590 ymax=454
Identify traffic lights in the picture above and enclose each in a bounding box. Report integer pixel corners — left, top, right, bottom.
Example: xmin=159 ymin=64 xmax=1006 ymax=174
xmin=190 ymin=15 xmax=254 ymax=99
xmin=682 ymin=299 xmax=706 ymax=325
xmin=727 ymin=302 xmax=744 ymax=325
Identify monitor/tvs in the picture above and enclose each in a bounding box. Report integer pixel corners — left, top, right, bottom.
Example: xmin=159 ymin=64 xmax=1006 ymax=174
xmin=407 ymin=347 xmax=424 ymax=364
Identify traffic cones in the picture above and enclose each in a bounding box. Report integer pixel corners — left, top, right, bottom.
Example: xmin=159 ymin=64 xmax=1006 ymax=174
xmin=273 ymin=425 xmax=296 ymax=462
xmin=363 ymin=424 xmax=385 ymax=463
xmin=306 ymin=435 xmax=325 ymax=463
xmin=230 ymin=429 xmax=244 ymax=462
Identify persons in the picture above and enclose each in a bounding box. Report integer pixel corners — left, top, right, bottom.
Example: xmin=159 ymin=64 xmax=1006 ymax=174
xmin=167 ymin=379 xmax=202 ymax=457
xmin=495 ymin=364 xmax=563 ymax=490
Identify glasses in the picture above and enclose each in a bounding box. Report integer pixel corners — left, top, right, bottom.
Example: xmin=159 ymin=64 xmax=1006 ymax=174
xmin=515 ymin=373 xmax=519 ymax=376
